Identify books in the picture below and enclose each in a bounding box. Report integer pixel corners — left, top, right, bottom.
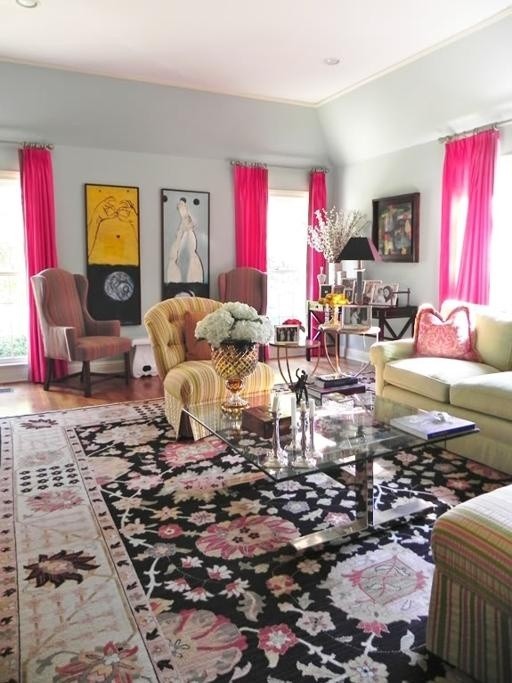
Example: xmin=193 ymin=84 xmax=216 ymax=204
xmin=306 ymin=385 xmax=366 ymax=399
xmin=390 ymin=410 xmax=476 ymax=440
xmin=314 ymin=373 xmax=359 ymax=388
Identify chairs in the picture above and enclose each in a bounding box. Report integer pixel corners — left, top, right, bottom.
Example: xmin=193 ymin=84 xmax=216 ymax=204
xmin=30 ymin=266 xmax=132 ymax=397
xmin=218 ymin=266 xmax=269 ymax=364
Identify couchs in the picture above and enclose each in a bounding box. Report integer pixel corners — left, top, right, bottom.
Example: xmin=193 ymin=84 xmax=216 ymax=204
xmin=142 ymin=297 xmax=274 ymax=439
xmin=425 ymin=483 xmax=512 ymax=683
xmin=367 ymin=298 xmax=512 ymax=476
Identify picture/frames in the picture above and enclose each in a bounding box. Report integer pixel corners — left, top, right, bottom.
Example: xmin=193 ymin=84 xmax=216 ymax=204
xmin=341 ymin=304 xmax=372 ymax=330
xmin=84 ymin=183 xmax=141 ymax=326
xmin=160 ymin=188 xmax=210 ymax=301
xmin=352 ymin=280 xmax=366 ymax=294
xmin=319 ymin=284 xmax=332 ymax=299
xmin=371 ymin=192 xmax=420 ymax=263
xmin=274 ymin=324 xmax=299 ymax=346
xmin=369 ymin=283 xmax=399 ymax=305
xmin=343 ymin=288 xmax=354 ymax=304
xmin=365 ymin=280 xmax=382 ymax=297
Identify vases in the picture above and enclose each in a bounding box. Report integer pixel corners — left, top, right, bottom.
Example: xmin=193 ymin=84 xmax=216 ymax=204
xmin=329 ymin=263 xmax=343 ymax=289
xmin=210 ymin=341 xmax=258 ymax=413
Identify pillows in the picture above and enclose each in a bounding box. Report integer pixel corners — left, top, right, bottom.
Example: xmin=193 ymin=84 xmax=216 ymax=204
xmin=412 ymin=303 xmax=482 ymax=362
xmin=184 ymin=311 xmax=211 ymax=362
xmin=474 ymin=309 xmax=511 ymax=371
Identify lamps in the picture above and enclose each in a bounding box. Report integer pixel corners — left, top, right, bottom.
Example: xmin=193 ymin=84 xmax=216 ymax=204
xmin=335 ymin=237 xmax=381 ymax=308
xmin=318 ymin=293 xmax=351 ymax=328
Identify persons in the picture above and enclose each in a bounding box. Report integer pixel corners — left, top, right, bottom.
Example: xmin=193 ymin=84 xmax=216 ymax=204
xmin=296 ymin=370 xmax=308 ymax=404
xmin=384 ymin=286 xmax=396 ymax=306
xmin=279 ymin=327 xmax=295 ymax=341
xmin=88 ymin=195 xmax=140 ymax=258
xmin=293 ymin=368 xmax=301 ymax=400
xmin=166 ymin=196 xmax=205 ymax=284
xmin=376 ymin=288 xmax=385 ymax=305
xmin=357 ymin=310 xmax=363 ymax=324
xmin=351 ymin=311 xmax=359 ymax=324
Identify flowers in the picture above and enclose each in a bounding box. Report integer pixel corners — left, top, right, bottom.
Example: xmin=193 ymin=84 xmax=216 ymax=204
xmin=283 ymin=319 xmax=305 ymax=332
xmin=305 ymin=207 xmax=370 ymax=263
xmin=193 ymin=301 xmax=272 ymax=350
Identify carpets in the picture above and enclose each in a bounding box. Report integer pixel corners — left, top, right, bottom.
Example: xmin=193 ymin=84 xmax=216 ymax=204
xmin=0 ymin=371 xmax=512 ymax=682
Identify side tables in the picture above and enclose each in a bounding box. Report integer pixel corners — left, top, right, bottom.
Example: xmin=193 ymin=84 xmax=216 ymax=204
xmin=268 ymin=340 xmax=321 ymax=392
xmin=129 ymin=338 xmax=158 ymax=378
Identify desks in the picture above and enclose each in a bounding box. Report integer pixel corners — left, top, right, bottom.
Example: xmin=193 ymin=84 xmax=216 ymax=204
xmin=306 ymin=300 xmax=418 ymax=363
xmin=323 ymin=323 xmax=380 ymax=377
